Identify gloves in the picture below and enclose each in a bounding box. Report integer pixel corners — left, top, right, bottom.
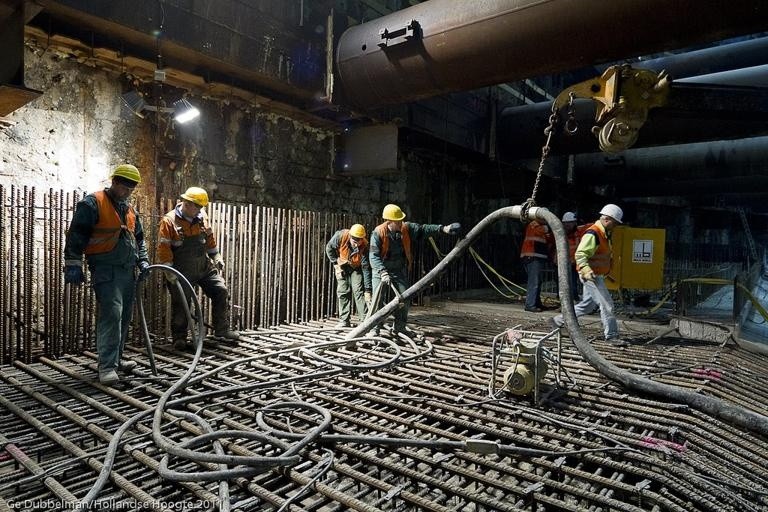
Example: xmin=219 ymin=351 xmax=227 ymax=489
xmin=137 ymin=260 xmax=151 ymax=281
xmin=212 ymin=252 xmax=225 ymax=271
xmin=581 ymin=265 xmax=596 ymax=282
xmin=333 ymin=265 xmax=346 ymax=280
xmin=364 ymin=291 xmax=372 ymax=304
xmin=64 ymin=259 xmax=87 ymax=287
xmin=379 ymin=269 xmax=393 ymax=286
xmin=163 ymin=268 xmax=178 ymax=285
xmin=442 ymin=223 xmax=461 ymax=235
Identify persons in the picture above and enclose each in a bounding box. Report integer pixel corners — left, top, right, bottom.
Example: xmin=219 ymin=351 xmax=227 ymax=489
xmin=64 ymin=163 xmax=150 ymax=384
xmin=368 ymin=203 xmax=461 ymax=334
xmin=550 ymin=204 xmax=627 ymax=346
xmin=519 ymin=208 xmax=553 ymax=312
xmin=326 ymin=223 xmax=371 ymax=328
xmin=157 ymin=186 xmax=241 ymax=350
xmin=553 ymin=211 xmax=582 ymax=303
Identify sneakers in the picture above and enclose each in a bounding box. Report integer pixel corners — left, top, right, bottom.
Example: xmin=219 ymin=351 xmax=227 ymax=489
xmin=550 ymin=317 xmax=570 ymax=337
xmin=214 ymin=328 xmax=240 ymax=340
xmin=98 ymin=369 xmax=120 ymax=386
xmin=118 ymin=359 xmax=137 ymax=373
xmin=605 ymin=334 xmax=626 ymax=346
xmin=524 ymin=304 xmax=549 ymax=313
xmin=335 ymin=320 xmax=416 ymax=341
xmin=172 ymin=337 xmax=187 ymax=351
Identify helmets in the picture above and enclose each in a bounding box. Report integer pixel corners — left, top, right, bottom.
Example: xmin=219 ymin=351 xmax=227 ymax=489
xmin=348 ymin=224 xmax=366 ymax=239
xmin=109 ymin=164 xmax=141 ymax=183
xmin=382 ymin=203 xmax=406 ymax=221
xmin=599 ymin=203 xmax=624 ymax=224
xmin=562 ymin=212 xmax=577 ymax=222
xmin=180 ymin=187 xmax=209 ymax=208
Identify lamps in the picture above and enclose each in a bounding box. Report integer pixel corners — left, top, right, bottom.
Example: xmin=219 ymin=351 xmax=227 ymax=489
xmin=120 ymin=39 xmax=202 ymax=127
